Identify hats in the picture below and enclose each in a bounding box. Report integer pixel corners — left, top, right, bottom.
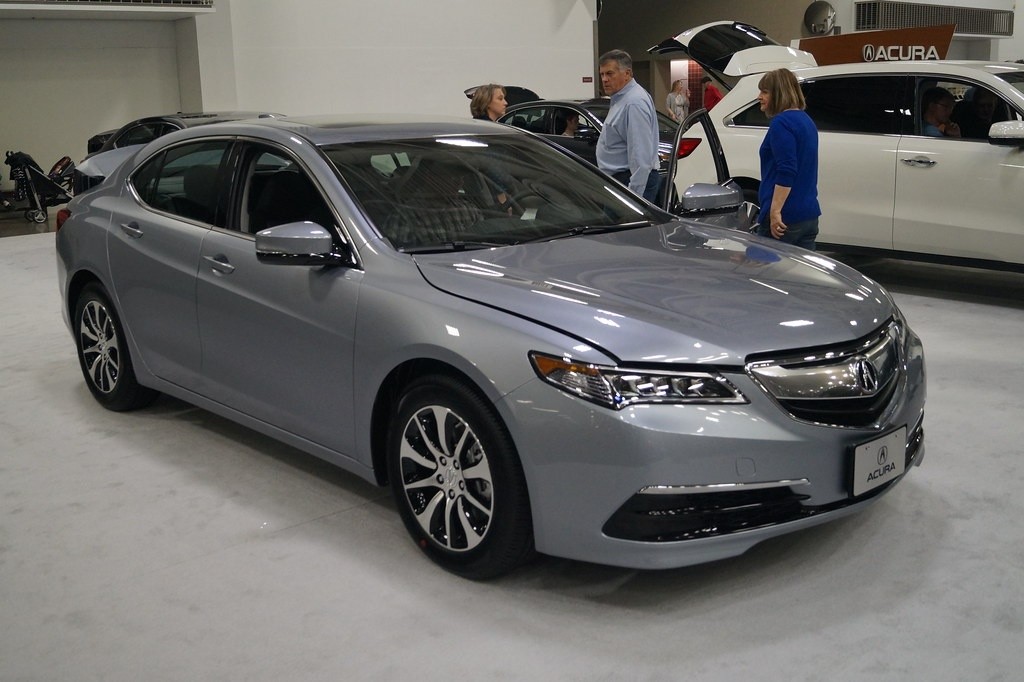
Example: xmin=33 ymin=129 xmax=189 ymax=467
xmin=700 ymin=76 xmax=713 ymax=83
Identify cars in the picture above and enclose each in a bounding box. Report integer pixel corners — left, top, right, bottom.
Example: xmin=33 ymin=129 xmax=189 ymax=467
xmin=78 ymin=112 xmax=289 ymax=174
xmin=646 ymin=20 xmax=1024 ymax=275
xmin=463 ymin=84 xmax=683 ymax=173
xmin=55 ymin=108 xmax=927 ymax=587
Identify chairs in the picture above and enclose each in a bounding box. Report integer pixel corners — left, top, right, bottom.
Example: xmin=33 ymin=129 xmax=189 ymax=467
xmin=512 ymin=115 xmax=546 ymax=134
xmin=364 ymin=164 xmax=427 ymax=215
xmin=397 ymin=162 xmax=482 ymax=243
xmin=171 ymin=163 xmax=221 ymax=225
xmin=245 ymin=169 xmax=323 ymax=244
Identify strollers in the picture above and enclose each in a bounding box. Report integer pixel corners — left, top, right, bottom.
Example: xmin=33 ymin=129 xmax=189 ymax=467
xmin=4 ymin=150 xmax=76 ymax=224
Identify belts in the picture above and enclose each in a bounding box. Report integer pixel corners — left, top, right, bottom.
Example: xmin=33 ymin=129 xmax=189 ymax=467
xmin=652 ymin=168 xmax=659 ymax=171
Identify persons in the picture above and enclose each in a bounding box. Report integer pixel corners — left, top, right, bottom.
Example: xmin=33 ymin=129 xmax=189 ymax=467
xmin=921 ymin=87 xmax=961 ymax=138
xmin=465 ymin=85 xmax=512 ymax=216
xmin=702 ymin=75 xmax=724 ymax=112
xmin=561 ymin=113 xmax=580 ymax=137
xmin=756 ymin=68 xmax=822 ymax=251
xmin=968 ymin=89 xmax=1008 ymax=138
xmin=665 ymin=80 xmax=691 ymax=124
xmin=596 ymin=49 xmax=661 ymax=224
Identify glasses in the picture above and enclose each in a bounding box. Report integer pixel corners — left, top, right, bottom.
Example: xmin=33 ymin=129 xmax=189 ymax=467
xmin=933 ymin=100 xmax=953 ymax=112
